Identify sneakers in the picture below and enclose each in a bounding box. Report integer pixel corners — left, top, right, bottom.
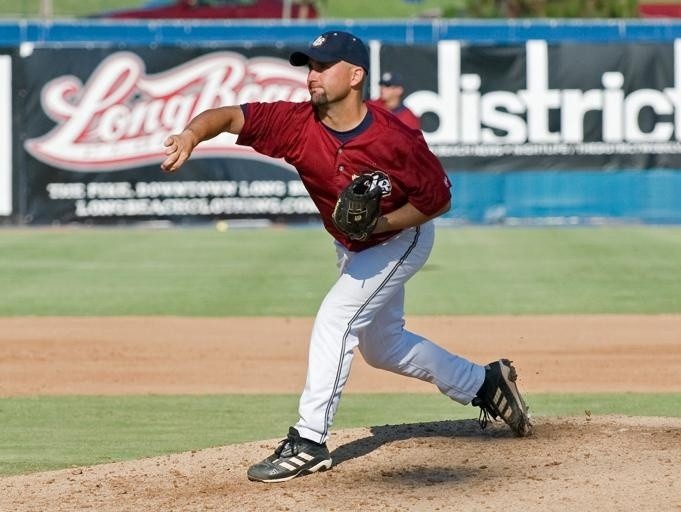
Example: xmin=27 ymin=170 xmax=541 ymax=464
xmin=249 ymin=426 xmax=333 ymax=484
xmin=472 ymin=359 xmax=534 ymax=436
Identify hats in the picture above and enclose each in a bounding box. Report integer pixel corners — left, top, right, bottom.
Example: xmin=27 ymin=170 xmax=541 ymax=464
xmin=379 ymin=71 xmax=405 ymax=86
xmin=290 ymin=31 xmax=368 ymax=71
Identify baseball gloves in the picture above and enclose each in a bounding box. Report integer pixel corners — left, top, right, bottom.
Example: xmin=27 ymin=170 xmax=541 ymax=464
xmin=330 ymin=174 xmax=383 ymax=241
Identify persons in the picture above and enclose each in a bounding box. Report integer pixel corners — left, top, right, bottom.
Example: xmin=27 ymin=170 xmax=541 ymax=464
xmin=158 ymin=28 xmax=530 ymax=487
xmin=372 ymin=70 xmax=420 ymax=132
